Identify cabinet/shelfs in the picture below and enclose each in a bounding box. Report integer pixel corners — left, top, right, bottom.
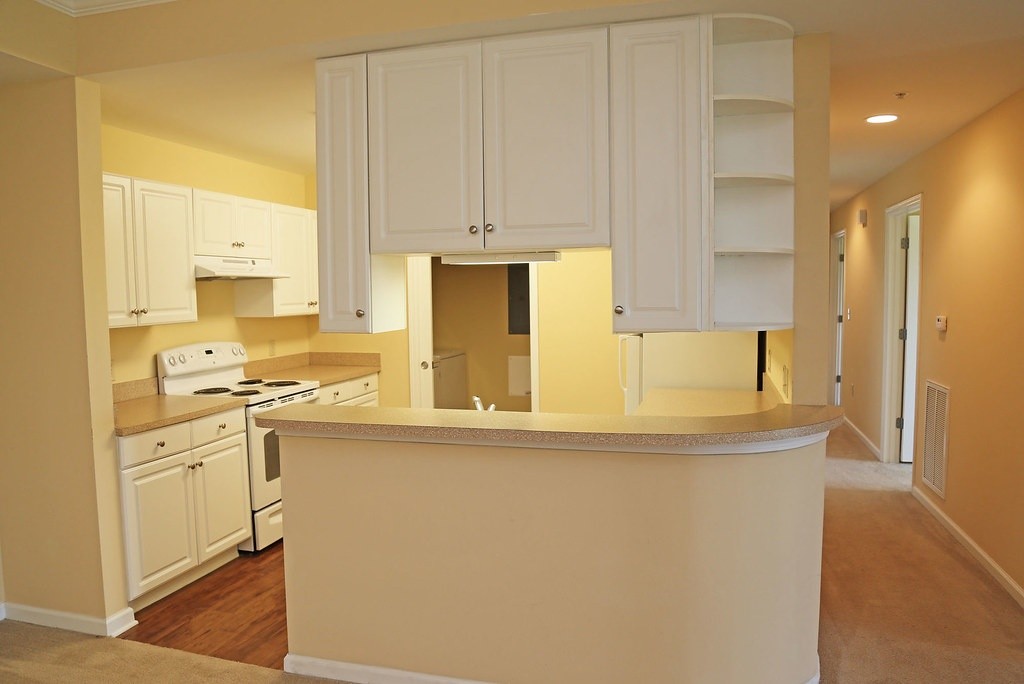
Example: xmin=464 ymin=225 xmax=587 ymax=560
xmin=231 ymin=203 xmax=319 ymax=318
xmin=366 ymin=23 xmax=612 ymax=258
xmin=710 ymin=13 xmax=798 ymax=334
xmin=192 ymin=187 xmax=271 ymax=260
xmin=609 ymin=13 xmax=709 ymax=334
xmin=320 ymin=372 xmax=379 ymax=407
xmin=312 ymin=51 xmax=406 ymax=334
xmin=102 ymin=170 xmax=199 ymax=329
xmin=116 ymin=405 xmax=252 ymax=613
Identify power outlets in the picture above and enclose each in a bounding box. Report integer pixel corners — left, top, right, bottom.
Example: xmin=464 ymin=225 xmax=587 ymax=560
xmin=268 ymin=339 xmax=276 ymax=356
